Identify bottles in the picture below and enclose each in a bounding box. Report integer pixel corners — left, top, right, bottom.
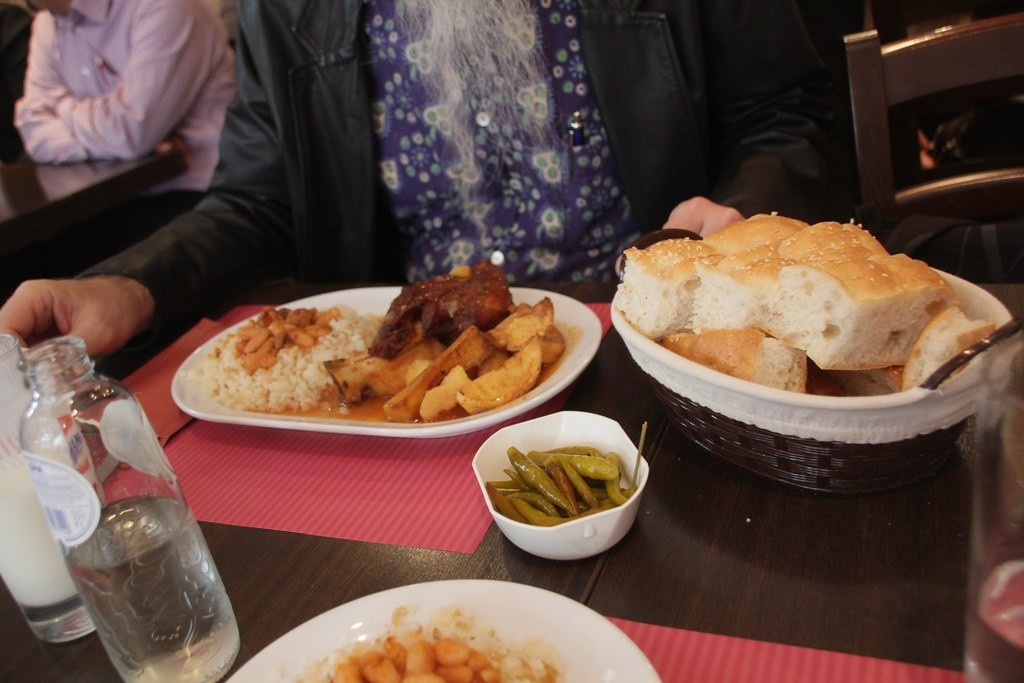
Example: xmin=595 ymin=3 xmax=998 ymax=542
xmin=15 ymin=335 xmax=242 ymax=683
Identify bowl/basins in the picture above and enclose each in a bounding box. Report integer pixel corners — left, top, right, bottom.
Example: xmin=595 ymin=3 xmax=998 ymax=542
xmin=602 ymin=237 xmax=1020 ymax=495
xmin=471 ymin=410 xmax=650 ymax=562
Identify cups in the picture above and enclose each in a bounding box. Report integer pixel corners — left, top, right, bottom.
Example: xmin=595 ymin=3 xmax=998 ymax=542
xmin=962 ymin=326 xmax=1024 ymax=683
xmin=0 ymin=333 xmax=114 ymax=642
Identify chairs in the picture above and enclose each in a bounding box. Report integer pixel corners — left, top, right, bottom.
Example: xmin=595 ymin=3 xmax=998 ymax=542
xmin=844 ymin=12 xmax=1024 ymax=222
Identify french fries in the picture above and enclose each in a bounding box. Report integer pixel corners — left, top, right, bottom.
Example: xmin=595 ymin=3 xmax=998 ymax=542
xmin=366 ymin=296 xmax=567 ymax=420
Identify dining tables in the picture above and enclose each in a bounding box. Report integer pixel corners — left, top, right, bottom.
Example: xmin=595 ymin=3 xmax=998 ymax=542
xmin=585 ymin=283 xmax=1024 ymax=683
xmin=1 ymin=274 xmax=669 ymax=683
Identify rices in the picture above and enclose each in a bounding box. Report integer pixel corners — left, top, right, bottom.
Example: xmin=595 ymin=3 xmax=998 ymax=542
xmin=182 ymin=303 xmax=384 ymax=414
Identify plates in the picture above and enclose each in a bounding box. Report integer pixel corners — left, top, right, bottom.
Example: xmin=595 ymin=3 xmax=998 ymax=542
xmin=169 ymin=283 xmax=604 ymax=438
xmin=224 ymin=580 xmax=667 ymax=683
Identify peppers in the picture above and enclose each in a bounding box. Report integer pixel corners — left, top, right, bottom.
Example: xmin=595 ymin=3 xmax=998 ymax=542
xmin=486 ymin=445 xmax=640 ymax=526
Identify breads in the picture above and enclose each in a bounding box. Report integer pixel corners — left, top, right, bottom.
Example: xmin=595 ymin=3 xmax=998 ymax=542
xmin=613 ymin=212 xmax=998 ymax=398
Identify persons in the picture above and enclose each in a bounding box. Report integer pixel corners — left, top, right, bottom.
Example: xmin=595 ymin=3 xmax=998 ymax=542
xmin=13 ymin=1 xmax=235 ymax=230
xmin=2 ymin=0 xmax=841 ymax=387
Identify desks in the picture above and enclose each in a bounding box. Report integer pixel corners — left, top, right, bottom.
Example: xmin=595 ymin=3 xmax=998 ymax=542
xmin=0 ymin=152 xmax=188 ymax=256
